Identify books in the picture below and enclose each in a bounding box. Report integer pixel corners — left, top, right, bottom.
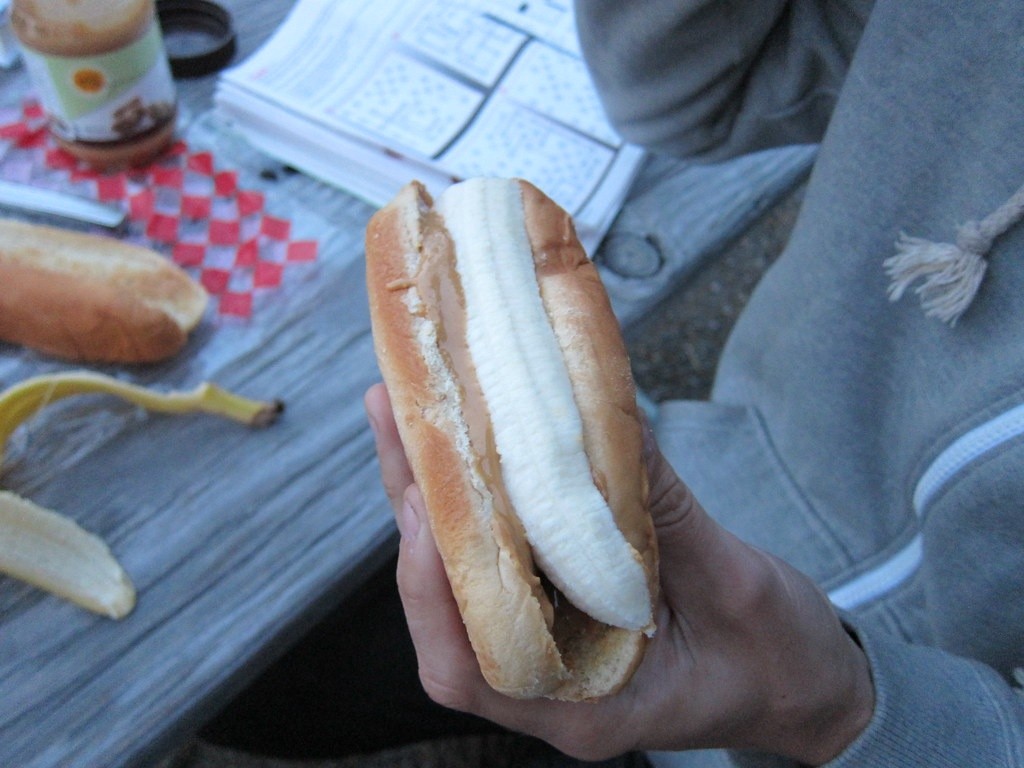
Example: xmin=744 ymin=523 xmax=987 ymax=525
xmin=207 ymin=1 xmax=645 ymax=261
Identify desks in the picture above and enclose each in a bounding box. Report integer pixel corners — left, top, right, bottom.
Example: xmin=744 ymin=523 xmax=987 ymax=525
xmin=1 ymin=2 xmax=833 ymax=767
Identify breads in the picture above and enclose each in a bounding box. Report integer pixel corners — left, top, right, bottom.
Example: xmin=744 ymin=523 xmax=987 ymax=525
xmin=362 ymin=178 xmax=660 ymax=704
xmin=1 ymin=214 xmax=214 ymax=369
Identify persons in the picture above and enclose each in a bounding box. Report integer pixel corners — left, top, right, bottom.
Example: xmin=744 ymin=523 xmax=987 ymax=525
xmin=365 ymin=1 xmax=1024 ymax=767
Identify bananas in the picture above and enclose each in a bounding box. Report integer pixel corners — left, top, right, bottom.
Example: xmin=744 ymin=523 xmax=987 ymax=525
xmin=436 ymin=176 xmax=653 ymax=631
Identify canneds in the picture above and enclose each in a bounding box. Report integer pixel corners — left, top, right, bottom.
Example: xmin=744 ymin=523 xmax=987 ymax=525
xmin=11 ymin=1 xmax=178 ymax=168
